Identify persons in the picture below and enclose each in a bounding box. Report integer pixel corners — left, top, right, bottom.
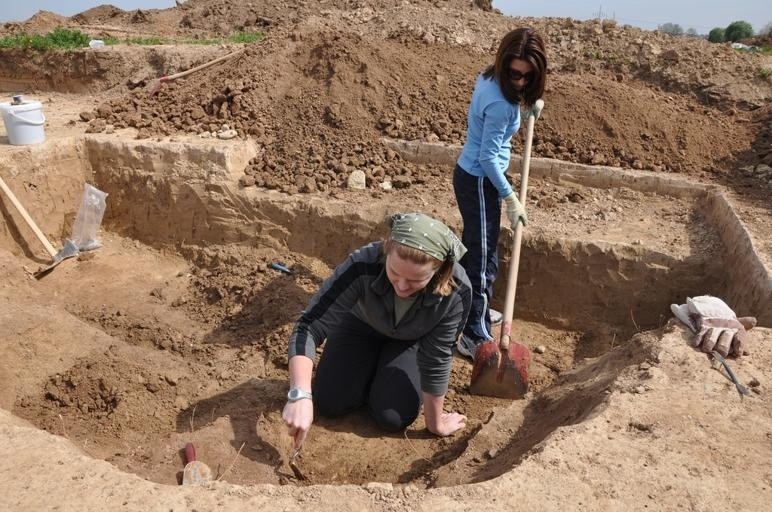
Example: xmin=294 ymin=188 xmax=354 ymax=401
xmin=281 ymin=212 xmax=474 ymax=451
xmin=452 ymin=26 xmax=547 ymax=362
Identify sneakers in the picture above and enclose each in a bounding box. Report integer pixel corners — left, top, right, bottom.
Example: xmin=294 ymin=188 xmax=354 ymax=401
xmin=457 ymin=307 xmax=506 ymax=361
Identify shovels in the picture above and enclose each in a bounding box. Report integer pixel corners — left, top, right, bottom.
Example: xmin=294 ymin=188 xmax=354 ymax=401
xmin=469 ymin=110 xmax=534 ymax=398
xmin=144 ymin=48 xmax=245 ymax=98
xmin=182 ymin=443 xmax=213 ymax=485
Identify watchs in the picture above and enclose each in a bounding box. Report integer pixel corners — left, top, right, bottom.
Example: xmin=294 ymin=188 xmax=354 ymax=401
xmin=286 ymin=388 xmax=314 ymax=402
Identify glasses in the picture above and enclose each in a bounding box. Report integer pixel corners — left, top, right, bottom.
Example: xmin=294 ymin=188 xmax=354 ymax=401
xmin=506 ymin=69 xmax=536 ymax=82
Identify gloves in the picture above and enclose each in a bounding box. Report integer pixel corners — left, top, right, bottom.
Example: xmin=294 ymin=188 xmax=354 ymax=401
xmin=503 ymin=191 xmax=528 ymax=230
xmin=670 ymin=294 xmax=757 ymax=360
xmin=531 ymin=99 xmax=544 ymax=121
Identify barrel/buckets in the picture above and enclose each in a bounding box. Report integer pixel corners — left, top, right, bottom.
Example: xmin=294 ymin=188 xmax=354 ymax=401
xmin=0 ymin=97 xmax=44 ymax=144
